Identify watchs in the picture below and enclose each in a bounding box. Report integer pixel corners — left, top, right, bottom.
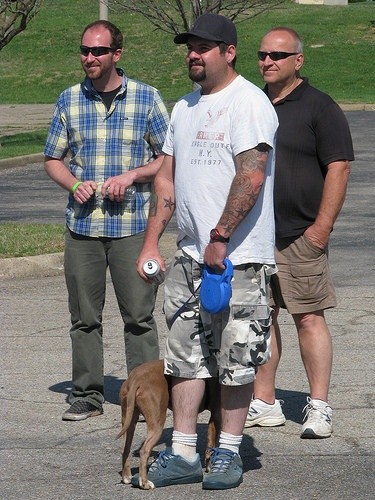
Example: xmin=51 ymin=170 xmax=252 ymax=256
xmin=210 ymin=229 xmax=230 ymax=243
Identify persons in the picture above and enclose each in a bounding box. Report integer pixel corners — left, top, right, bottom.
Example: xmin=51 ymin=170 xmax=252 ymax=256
xmin=44 ymin=20 xmax=171 ymax=421
xmin=244 ymin=27 xmax=355 ymax=438
xmin=131 ymin=14 xmax=279 ymax=491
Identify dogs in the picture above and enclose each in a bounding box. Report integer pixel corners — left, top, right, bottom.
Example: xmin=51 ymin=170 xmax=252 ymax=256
xmin=112 ymin=357 xmax=220 ymax=490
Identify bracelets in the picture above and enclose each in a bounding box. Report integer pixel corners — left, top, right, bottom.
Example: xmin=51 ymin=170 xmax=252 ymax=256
xmin=71 ymin=182 xmax=82 ymax=192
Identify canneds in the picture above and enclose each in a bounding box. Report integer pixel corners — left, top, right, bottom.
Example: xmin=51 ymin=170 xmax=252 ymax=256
xmin=143 ymin=259 xmax=165 ymax=285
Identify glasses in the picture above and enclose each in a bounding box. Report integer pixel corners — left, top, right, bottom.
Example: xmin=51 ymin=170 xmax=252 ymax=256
xmin=79 ymin=44 xmax=113 ymax=56
xmin=257 ymin=50 xmax=298 ymax=61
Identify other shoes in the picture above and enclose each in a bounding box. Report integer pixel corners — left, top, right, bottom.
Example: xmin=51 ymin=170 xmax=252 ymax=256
xmin=137 ymin=413 xmax=146 ymax=422
xmin=61 ymin=399 xmax=103 ymax=422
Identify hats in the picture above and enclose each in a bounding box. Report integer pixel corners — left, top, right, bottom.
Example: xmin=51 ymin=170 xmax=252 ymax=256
xmin=172 ymin=12 xmax=238 ymax=48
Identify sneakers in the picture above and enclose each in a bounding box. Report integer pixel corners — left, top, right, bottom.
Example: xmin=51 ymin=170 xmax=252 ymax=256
xmin=244 ymin=393 xmax=286 ymax=428
xmin=299 ymin=395 xmax=334 ymax=439
xmin=130 ymin=447 xmax=203 ymax=489
xmin=201 ymin=446 xmax=244 ymax=490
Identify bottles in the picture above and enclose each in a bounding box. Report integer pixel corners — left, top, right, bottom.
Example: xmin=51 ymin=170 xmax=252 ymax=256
xmin=142 ymin=259 xmax=166 ymax=285
xmin=94 ymin=183 xmax=136 ymax=200
